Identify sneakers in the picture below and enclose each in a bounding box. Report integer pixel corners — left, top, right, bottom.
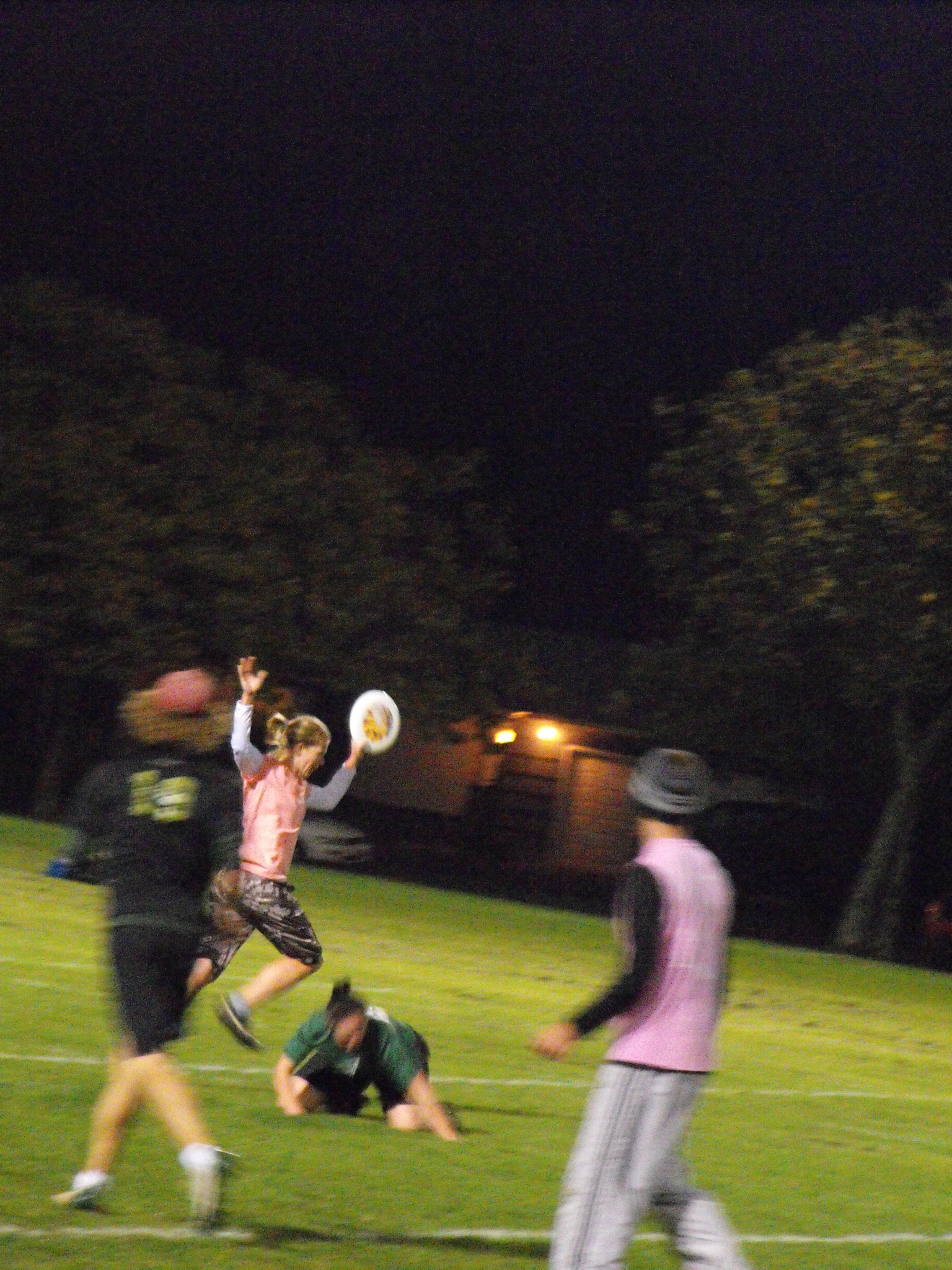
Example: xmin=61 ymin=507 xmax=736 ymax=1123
xmin=188 ymin=1153 xmax=234 ymax=1226
xmin=55 ymin=1181 xmax=105 ymax=1214
xmin=217 ymin=995 xmax=271 ymax=1052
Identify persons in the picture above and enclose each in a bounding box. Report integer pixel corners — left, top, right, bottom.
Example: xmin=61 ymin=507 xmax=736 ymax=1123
xmin=50 ymin=670 xmax=243 ymax=1233
xmin=187 ymin=655 xmax=368 ymax=1054
xmin=272 ymin=976 xmax=468 ymax=1140
xmin=529 ymin=751 xmax=754 ymax=1270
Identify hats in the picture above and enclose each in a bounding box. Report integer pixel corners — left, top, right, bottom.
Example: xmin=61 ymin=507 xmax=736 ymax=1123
xmin=155 ymin=669 xmax=215 ymax=715
xmin=628 ymin=747 xmax=723 ymax=814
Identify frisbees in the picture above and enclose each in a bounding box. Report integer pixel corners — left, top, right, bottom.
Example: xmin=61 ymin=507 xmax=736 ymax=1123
xmin=348 ymin=688 xmax=402 ymax=754
